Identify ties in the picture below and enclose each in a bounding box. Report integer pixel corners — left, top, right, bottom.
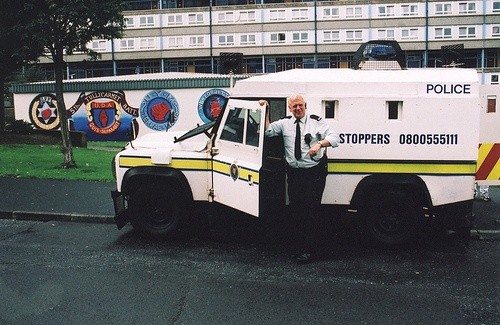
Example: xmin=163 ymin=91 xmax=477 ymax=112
xmin=295 ymin=118 xmax=301 ymax=160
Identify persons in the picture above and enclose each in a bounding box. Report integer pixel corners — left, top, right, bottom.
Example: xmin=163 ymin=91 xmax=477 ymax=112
xmin=258 ymin=95 xmax=341 ymax=263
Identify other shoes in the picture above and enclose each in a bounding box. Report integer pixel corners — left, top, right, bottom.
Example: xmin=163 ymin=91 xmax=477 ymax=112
xmin=289 ymin=248 xmax=321 ymax=263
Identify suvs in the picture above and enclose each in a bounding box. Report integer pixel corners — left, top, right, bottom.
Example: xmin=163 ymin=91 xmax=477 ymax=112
xmin=110 ymin=38 xmax=481 ymax=244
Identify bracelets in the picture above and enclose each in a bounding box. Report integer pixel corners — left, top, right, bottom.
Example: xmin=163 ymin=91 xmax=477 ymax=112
xmin=317 ymin=141 xmax=325 ymax=148
